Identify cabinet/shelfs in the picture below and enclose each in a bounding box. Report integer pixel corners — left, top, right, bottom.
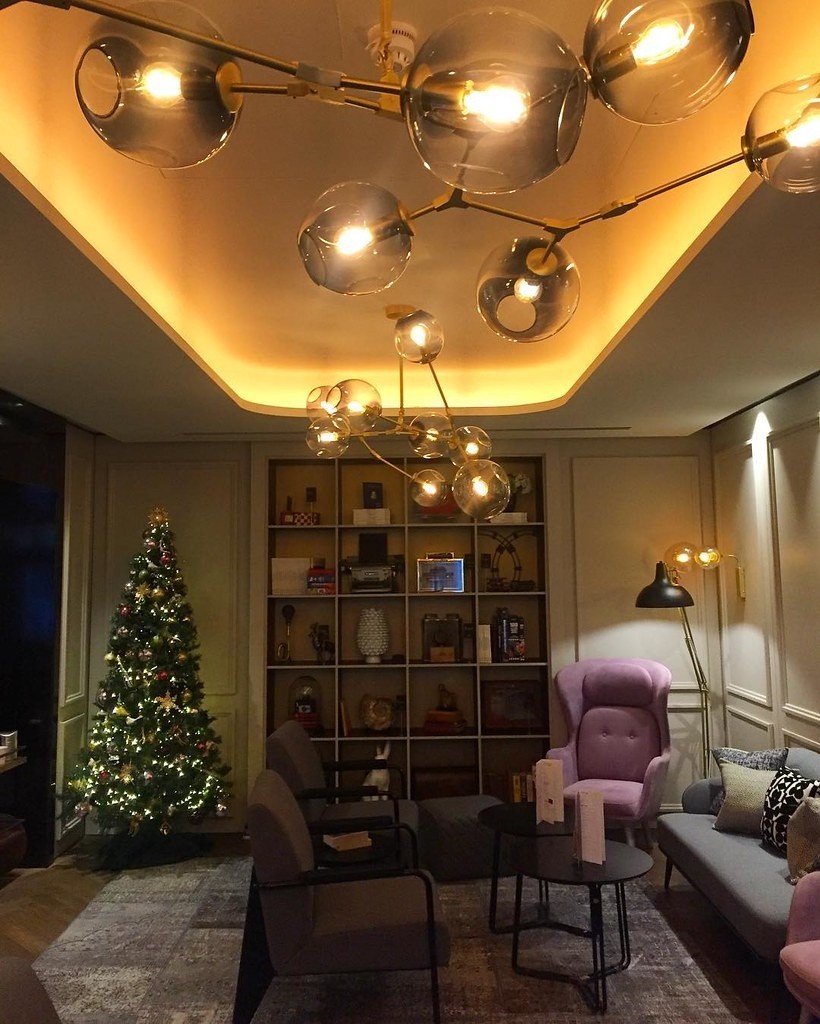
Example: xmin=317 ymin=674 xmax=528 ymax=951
xmin=264 ymin=454 xmax=554 ymax=802
xmin=0 ymin=758 xmax=26 ymax=877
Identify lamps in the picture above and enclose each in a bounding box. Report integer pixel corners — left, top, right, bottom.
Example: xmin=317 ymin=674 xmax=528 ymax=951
xmin=0 ymin=1 xmax=820 ymax=517
xmin=635 ymin=560 xmax=712 ymax=780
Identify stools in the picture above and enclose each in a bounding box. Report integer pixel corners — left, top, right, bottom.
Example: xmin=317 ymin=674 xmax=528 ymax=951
xmin=416 ymin=795 xmax=516 ymax=880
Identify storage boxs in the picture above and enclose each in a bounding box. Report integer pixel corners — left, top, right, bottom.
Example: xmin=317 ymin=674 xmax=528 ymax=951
xmin=481 ymin=678 xmax=548 ymax=733
xmin=271 ymin=557 xmax=337 ymax=594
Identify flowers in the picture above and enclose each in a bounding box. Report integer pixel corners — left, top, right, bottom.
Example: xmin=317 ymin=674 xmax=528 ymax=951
xmin=508 ymin=471 xmax=532 ymax=495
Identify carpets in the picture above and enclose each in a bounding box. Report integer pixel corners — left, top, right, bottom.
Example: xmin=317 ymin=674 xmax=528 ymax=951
xmin=30 ymin=853 xmax=753 ymax=1024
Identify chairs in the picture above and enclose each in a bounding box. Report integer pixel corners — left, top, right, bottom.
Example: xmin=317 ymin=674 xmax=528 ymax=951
xmin=246 ymin=769 xmax=450 ymax=1024
xmin=544 ymin=659 xmax=671 ymax=847
xmin=267 ymin=719 xmax=418 ymax=861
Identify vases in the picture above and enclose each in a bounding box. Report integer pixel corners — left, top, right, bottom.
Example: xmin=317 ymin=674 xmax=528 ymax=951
xmin=503 ymin=493 xmax=515 ymax=513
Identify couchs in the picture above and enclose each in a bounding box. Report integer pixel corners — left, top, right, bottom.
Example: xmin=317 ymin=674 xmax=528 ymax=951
xmin=654 ymin=748 xmax=820 ymax=962
xmin=779 ymin=871 xmax=820 ymax=1024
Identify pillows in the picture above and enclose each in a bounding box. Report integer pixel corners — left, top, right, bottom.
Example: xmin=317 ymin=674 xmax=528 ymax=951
xmin=711 ymin=747 xmax=790 ymax=815
xmin=786 ymin=796 xmax=820 ymax=884
xmin=711 ymin=757 xmax=800 ymax=835
xmin=760 ymin=765 xmax=820 ymax=853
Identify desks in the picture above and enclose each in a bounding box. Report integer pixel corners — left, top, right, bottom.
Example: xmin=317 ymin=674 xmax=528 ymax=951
xmin=511 ymin=835 xmax=655 ymax=1012
xmin=478 ymin=802 xmax=594 ymax=939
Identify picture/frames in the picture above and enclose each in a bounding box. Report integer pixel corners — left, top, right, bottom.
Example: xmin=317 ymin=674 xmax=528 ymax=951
xmin=417 ymin=558 xmax=465 ymax=593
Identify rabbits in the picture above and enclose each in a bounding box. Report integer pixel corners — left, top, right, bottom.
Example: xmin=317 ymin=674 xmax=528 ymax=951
xmin=362 ymin=743 xmax=392 ymax=802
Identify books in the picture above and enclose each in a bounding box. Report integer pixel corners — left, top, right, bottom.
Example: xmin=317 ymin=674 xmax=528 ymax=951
xmin=513 ymin=771 xmax=533 ymax=803
xmin=536 ymin=759 xmax=564 ymax=824
xmin=574 ymin=788 xmax=606 ymax=866
